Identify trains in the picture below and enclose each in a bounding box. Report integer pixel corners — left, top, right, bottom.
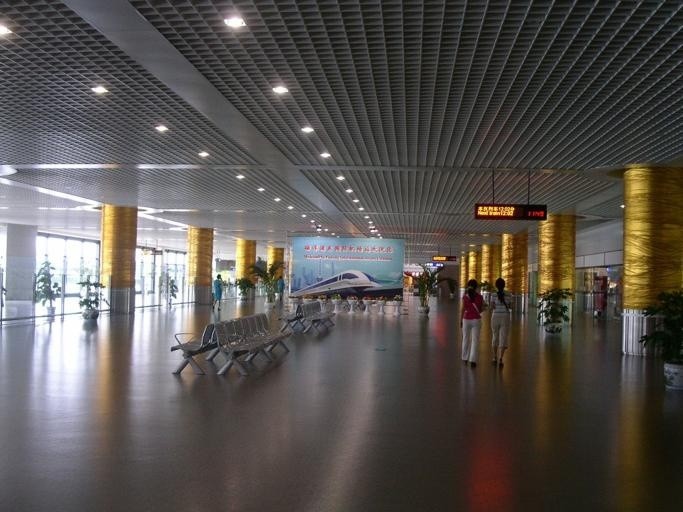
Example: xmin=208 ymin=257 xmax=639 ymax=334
xmin=290 ymin=270 xmax=382 ymax=298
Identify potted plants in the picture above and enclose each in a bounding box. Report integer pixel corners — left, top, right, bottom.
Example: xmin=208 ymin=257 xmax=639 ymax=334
xmin=233 ymin=257 xmax=281 ymax=314
xmin=638 ymin=289 xmax=682 ymax=390
xmin=34 ymin=261 xmax=112 ymax=321
xmin=535 ymin=288 xmax=573 ymax=338
xmin=404 ymin=263 xmax=456 ymax=317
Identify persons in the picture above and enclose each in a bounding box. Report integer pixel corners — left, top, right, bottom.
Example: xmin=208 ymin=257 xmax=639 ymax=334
xmin=211 ymin=274 xmax=223 ymax=312
xmin=459 ymin=279 xmax=484 ymax=368
xmin=489 ymin=278 xmax=512 ymax=368
xmin=277 ymin=276 xmax=285 ymax=300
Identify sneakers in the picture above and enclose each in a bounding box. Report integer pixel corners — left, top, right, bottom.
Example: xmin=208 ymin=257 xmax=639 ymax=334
xmin=493 ymin=358 xmax=504 ymax=367
xmin=464 ymin=360 xmax=477 ymax=368
xmin=213 ymin=306 xmax=221 ymax=311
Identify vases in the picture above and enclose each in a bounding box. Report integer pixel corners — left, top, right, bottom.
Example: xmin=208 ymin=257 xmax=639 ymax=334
xmin=304 ymin=299 xmax=402 ymax=316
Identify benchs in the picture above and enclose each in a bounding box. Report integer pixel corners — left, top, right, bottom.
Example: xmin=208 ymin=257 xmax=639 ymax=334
xmin=171 ymin=301 xmax=336 ymax=377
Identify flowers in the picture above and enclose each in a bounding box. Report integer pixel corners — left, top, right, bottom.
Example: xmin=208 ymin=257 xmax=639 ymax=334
xmin=303 ymin=293 xmax=403 ymax=300
